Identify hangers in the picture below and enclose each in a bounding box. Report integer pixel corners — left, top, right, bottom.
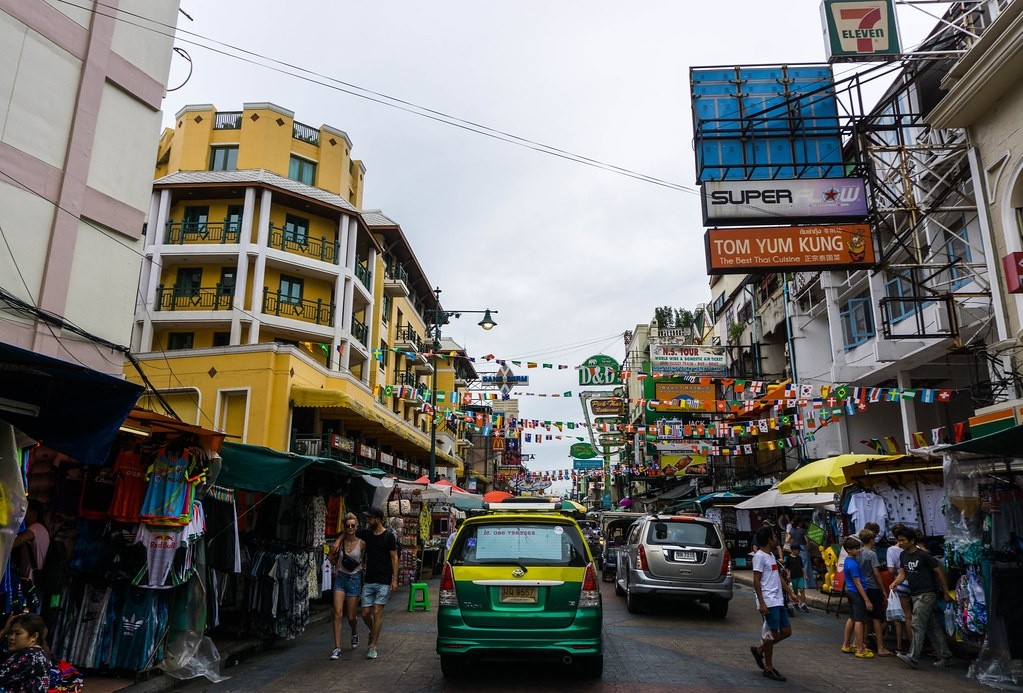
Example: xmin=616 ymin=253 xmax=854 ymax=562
xmin=257 ymin=538 xmax=304 ymax=555
xmin=849 ymin=477 xmax=934 ymax=494
xmin=37 ymin=436 xmax=194 ymax=601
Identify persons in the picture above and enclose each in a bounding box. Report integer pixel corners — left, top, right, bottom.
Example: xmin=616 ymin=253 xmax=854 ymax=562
xmin=752 ymin=519 xmax=795 ymax=617
xmin=446 ymin=518 xmax=465 ymax=552
xmin=889 ymin=526 xmax=952 ymax=668
xmin=13 ymin=498 xmax=50 ymax=580
xmin=0 ymin=612 xmax=54 ymax=693
xmin=784 ymin=544 xmax=810 ymax=613
xmin=582 ymin=522 xmax=594 ymax=544
xmin=840 ymin=537 xmax=875 ymax=657
xmin=838 ymin=520 xmax=930 ymax=656
xmin=778 ymin=507 xmax=818 ymax=589
xmin=330 ymin=505 xmax=399 ymax=659
xmin=750 ymin=525 xmax=798 ymax=682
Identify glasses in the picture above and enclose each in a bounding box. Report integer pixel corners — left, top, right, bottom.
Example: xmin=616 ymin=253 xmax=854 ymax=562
xmin=345 ymin=524 xmax=357 ymax=529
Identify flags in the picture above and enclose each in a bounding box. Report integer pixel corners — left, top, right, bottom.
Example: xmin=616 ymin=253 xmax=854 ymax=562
xmin=318 ymin=343 xmax=965 ymax=482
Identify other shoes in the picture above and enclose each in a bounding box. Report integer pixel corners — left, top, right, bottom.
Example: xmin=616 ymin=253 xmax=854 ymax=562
xmin=897 ymin=653 xmax=918 ymax=667
xmin=896 ymin=648 xmax=906 ymax=654
xmin=878 ymin=650 xmax=893 ymax=656
xmin=751 ymin=646 xmax=766 ymax=670
xmin=793 ymin=603 xmax=800 ymax=609
xmin=762 ymin=668 xmax=787 ymax=682
xmin=802 ymin=604 xmax=809 ymax=613
xmin=934 ymin=656 xmax=953 ymax=666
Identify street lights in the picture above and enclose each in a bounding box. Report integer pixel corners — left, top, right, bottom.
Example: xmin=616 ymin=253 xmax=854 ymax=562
xmin=514 ymin=454 xmax=537 ymax=496
xmin=424 ymin=284 xmax=498 ymax=482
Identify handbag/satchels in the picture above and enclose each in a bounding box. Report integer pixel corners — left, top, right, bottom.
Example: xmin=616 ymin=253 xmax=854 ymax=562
xmin=886 ymin=589 xmax=905 ymax=622
xmin=761 ymin=615 xmax=774 ymax=644
xmin=944 ymin=602 xmax=957 ymax=636
xmin=341 ymin=537 xmax=362 ymax=573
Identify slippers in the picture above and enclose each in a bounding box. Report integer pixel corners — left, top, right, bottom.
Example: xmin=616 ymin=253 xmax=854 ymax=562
xmin=842 ymin=644 xmax=856 ymax=652
xmin=855 ymin=649 xmax=874 ymax=657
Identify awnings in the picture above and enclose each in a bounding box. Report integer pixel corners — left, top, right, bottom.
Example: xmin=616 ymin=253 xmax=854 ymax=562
xmin=640 ymin=495 xmax=658 ymax=504
xmin=123 ymin=402 xmax=241 ymax=453
xmin=390 ymin=473 xmax=484 ymax=510
xmin=657 ymin=481 xmax=702 ymax=498
xmin=733 ymin=482 xmax=839 ymax=508
xmin=932 ymin=423 xmax=1023 ymax=455
xmin=717 ymin=485 xmax=773 ymax=493
xmin=1 ymin=340 xmax=147 ymax=467
xmin=215 ymin=442 xmax=386 ymax=497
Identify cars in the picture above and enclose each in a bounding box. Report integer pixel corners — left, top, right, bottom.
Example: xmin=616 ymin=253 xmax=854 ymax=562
xmin=614 ymin=513 xmax=733 ymax=617
xmin=436 ymin=499 xmax=603 ymax=679
xmin=558 ymin=511 xmax=645 ymax=583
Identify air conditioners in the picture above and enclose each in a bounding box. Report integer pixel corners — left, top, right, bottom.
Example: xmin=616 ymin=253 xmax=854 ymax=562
xmin=295 ymin=439 xmax=322 ymax=455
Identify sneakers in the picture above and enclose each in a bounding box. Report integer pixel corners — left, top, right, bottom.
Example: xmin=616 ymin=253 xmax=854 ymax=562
xmin=366 ymin=647 xmax=377 ymax=658
xmin=368 ymin=631 xmax=372 ymax=646
xmin=330 ymin=647 xmax=342 ymax=659
xmin=351 ymin=633 xmax=359 ymax=648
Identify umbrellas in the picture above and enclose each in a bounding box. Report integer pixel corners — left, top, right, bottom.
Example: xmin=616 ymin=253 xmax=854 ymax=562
xmin=781 ymin=452 xmax=909 ymax=494
xmin=557 ymin=500 xmax=587 ymax=513
xmin=483 ymin=489 xmax=515 ymax=503
xmin=677 ymin=490 xmax=746 ymax=506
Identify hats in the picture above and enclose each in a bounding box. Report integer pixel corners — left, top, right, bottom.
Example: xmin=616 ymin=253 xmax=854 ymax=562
xmin=790 ymin=543 xmax=804 ymax=551
xmin=454 ymin=518 xmax=465 ymax=529
xmin=362 ymin=507 xmax=384 ymax=521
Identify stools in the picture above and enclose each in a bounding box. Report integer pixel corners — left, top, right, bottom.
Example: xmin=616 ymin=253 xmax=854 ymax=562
xmin=407 ymin=583 xmax=430 ymax=612
